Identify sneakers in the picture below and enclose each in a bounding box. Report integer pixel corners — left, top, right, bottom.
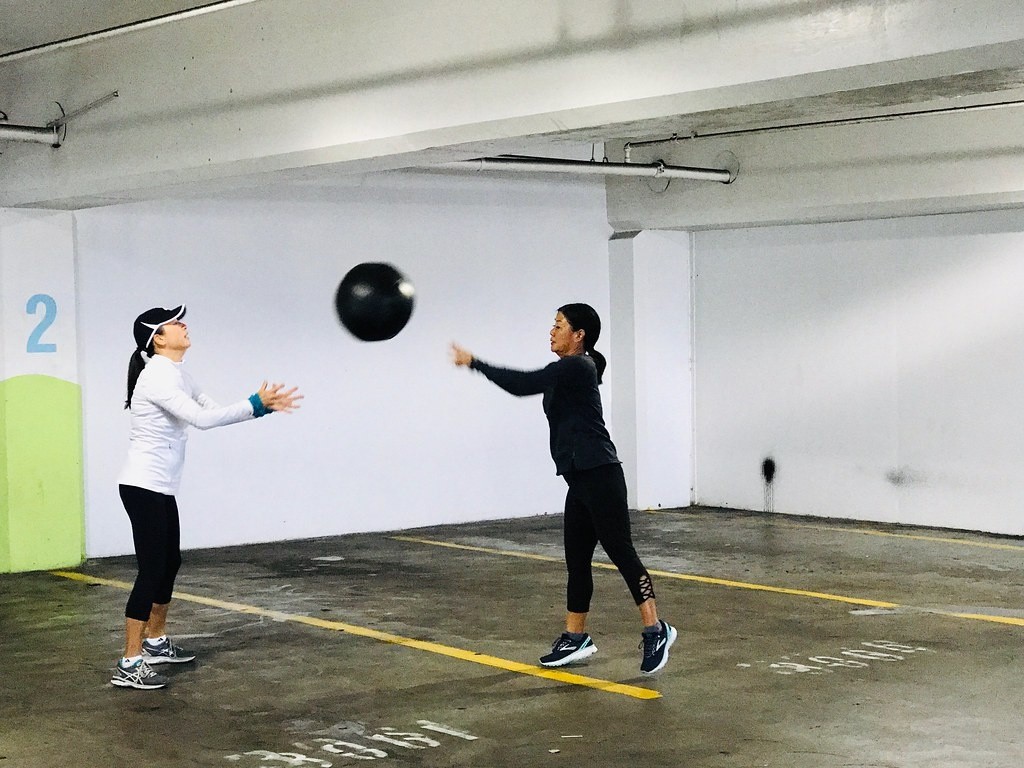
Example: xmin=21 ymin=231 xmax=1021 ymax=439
xmin=540 ymin=633 xmax=598 ymax=667
xmin=110 ymin=658 xmax=168 ymax=689
xmin=139 ymin=637 xmax=197 ymax=663
xmin=639 ymin=619 xmax=676 ymax=671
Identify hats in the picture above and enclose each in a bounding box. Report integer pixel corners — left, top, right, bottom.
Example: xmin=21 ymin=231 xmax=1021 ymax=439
xmin=133 ymin=303 xmax=186 ymax=349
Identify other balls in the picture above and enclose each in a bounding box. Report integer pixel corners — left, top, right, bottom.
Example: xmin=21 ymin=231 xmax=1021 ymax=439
xmin=335 ymin=262 xmax=415 ymax=341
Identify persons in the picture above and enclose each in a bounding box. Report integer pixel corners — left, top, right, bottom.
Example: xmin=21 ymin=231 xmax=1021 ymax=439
xmin=110 ymin=305 xmax=305 ymax=690
xmin=453 ymin=303 xmax=678 ymax=674
